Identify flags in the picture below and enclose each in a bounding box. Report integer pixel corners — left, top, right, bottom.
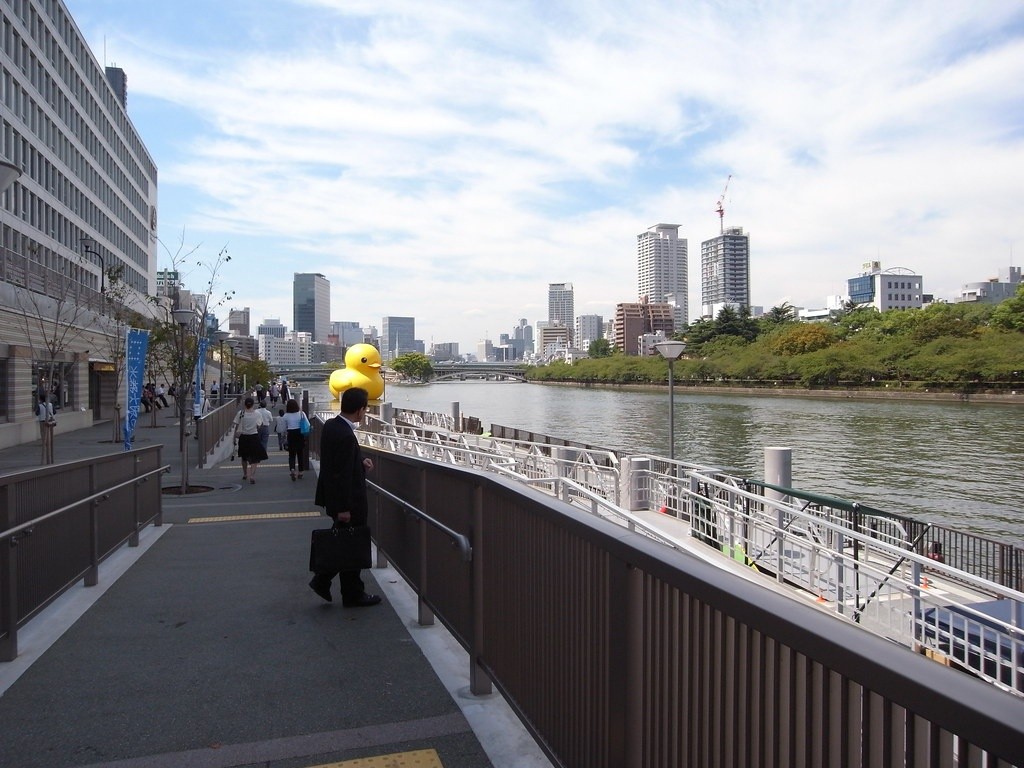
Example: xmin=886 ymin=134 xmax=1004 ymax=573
xmin=193 ymin=336 xmax=209 ymax=417
xmin=124 ymin=330 xmax=147 ymax=451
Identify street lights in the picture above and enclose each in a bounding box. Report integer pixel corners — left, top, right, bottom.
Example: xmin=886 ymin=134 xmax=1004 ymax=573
xmin=170 ymin=309 xmax=197 ymax=449
xmin=225 ymin=339 xmax=239 ymax=390
xmin=215 ymin=330 xmax=232 ymax=407
xmin=653 ymin=339 xmax=688 ymax=475
xmin=233 ymin=348 xmax=243 ymax=393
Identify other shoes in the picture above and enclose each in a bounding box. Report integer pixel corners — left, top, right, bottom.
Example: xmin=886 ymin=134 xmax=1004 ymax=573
xmin=290 ymin=470 xmax=296 ymax=481
xmin=298 ymin=474 xmax=304 ymax=479
xmin=243 ymin=475 xmax=248 ymax=480
xmin=194 ymin=436 xmax=198 ymax=439
xmin=165 ymin=406 xmax=169 ymax=407
xmin=160 ymin=407 xmax=164 ymax=410
xmin=250 ymin=478 xmax=256 ymax=484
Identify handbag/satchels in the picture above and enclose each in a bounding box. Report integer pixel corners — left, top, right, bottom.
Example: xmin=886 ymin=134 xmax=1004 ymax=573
xmin=235 ymin=418 xmax=242 ymax=437
xmin=309 ymin=520 xmax=372 ymax=570
xmin=300 ymin=412 xmax=311 ymax=437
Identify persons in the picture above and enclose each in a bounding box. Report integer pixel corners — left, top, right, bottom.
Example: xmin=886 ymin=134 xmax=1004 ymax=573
xmin=282 ymin=398 xmax=311 ymax=482
xmin=35 ymin=394 xmax=57 ymax=445
xmin=255 ymin=380 xmax=288 ymax=450
xmin=141 ymin=380 xmax=237 ymax=440
xmin=308 ymin=387 xmax=381 ymax=607
xmin=233 ymin=397 xmax=268 ymax=485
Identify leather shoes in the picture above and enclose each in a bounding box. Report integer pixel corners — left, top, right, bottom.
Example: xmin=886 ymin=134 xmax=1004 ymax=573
xmin=344 ymin=594 xmax=382 ymax=607
xmin=309 ymin=575 xmax=332 ymax=602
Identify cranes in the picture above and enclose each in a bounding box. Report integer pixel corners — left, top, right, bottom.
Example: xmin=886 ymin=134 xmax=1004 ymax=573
xmin=714 ymin=173 xmax=734 ymax=234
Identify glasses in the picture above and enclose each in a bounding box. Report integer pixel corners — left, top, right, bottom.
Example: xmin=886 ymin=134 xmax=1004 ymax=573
xmin=364 ymin=407 xmax=370 ymax=413
